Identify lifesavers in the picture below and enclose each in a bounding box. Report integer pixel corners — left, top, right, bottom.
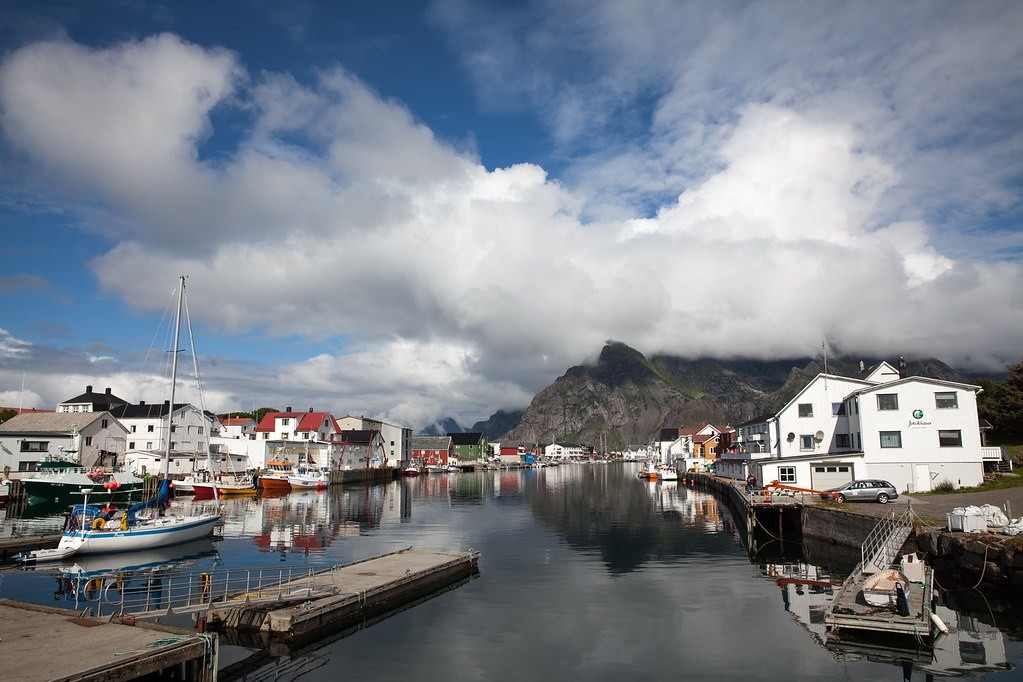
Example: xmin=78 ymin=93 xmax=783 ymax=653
xmin=87 ymin=580 xmax=100 ymax=591
xmin=92 ymin=518 xmax=106 ymax=529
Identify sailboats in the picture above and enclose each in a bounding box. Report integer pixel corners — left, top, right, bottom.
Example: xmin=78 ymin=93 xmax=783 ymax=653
xmin=57 ymin=275 xmax=221 ymax=555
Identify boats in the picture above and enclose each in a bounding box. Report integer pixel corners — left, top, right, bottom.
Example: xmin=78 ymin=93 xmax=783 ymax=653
xmin=402 ymin=459 xmax=420 ymax=476
xmin=425 ymin=455 xmax=461 ymax=473
xmin=170 ymin=435 xmax=330 ymax=500
xmin=638 ymin=446 xmax=678 ymax=480
xmin=10 ymin=548 xmax=78 ymax=566
xmin=2 ymin=423 xmax=164 ymax=506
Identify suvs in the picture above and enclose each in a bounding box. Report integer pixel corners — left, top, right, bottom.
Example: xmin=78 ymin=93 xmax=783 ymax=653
xmin=819 ymin=480 xmax=899 ymax=504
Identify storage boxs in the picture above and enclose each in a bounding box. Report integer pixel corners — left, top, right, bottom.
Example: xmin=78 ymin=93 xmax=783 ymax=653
xmin=945 ymin=512 xmax=988 ymax=533
xmin=902 ymin=559 xmax=925 ymax=584
